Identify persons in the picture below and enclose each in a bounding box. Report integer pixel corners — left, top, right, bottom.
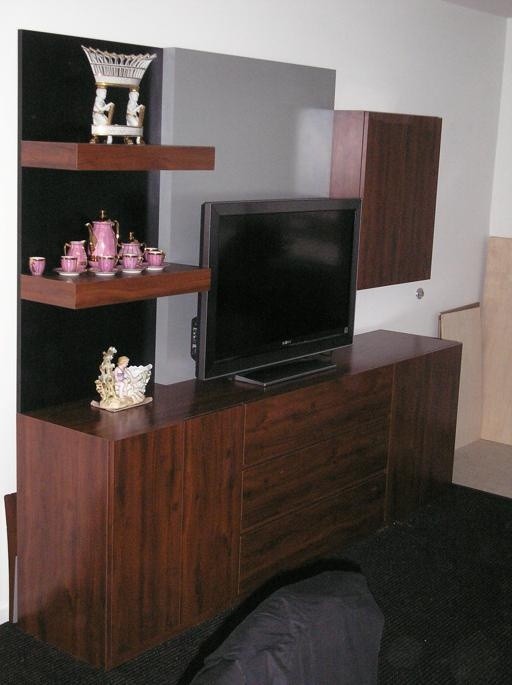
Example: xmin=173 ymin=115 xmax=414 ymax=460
xmin=92 ymin=88 xmax=114 ymax=125
xmin=113 ymin=356 xmax=129 ymax=399
xmin=126 ymin=91 xmax=143 ymax=127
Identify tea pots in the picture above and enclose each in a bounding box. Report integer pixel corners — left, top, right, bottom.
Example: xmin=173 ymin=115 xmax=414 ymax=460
xmin=64 ymin=210 xmax=147 ymax=272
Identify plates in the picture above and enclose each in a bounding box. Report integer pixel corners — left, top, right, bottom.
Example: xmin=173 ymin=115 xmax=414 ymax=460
xmin=55 ymin=265 xmax=170 ymax=276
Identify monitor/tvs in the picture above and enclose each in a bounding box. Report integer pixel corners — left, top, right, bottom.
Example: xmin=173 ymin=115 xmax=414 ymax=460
xmin=190 ymin=197 xmax=362 ymax=387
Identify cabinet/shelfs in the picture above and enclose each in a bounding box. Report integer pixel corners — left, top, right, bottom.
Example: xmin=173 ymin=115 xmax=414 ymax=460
xmin=11 ymin=328 xmax=464 ymax=676
xmin=330 ymin=107 xmax=443 ymax=292
xmin=18 ymin=139 xmax=216 ymax=312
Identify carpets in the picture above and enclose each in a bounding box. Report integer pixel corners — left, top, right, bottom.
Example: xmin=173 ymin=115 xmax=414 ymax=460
xmin=0 ymin=485 xmax=512 ymax=684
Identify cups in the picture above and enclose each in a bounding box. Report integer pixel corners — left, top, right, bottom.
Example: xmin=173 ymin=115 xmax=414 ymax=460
xmin=61 ymin=248 xmax=165 ymax=272
xmin=29 ymin=257 xmax=46 ymax=275
